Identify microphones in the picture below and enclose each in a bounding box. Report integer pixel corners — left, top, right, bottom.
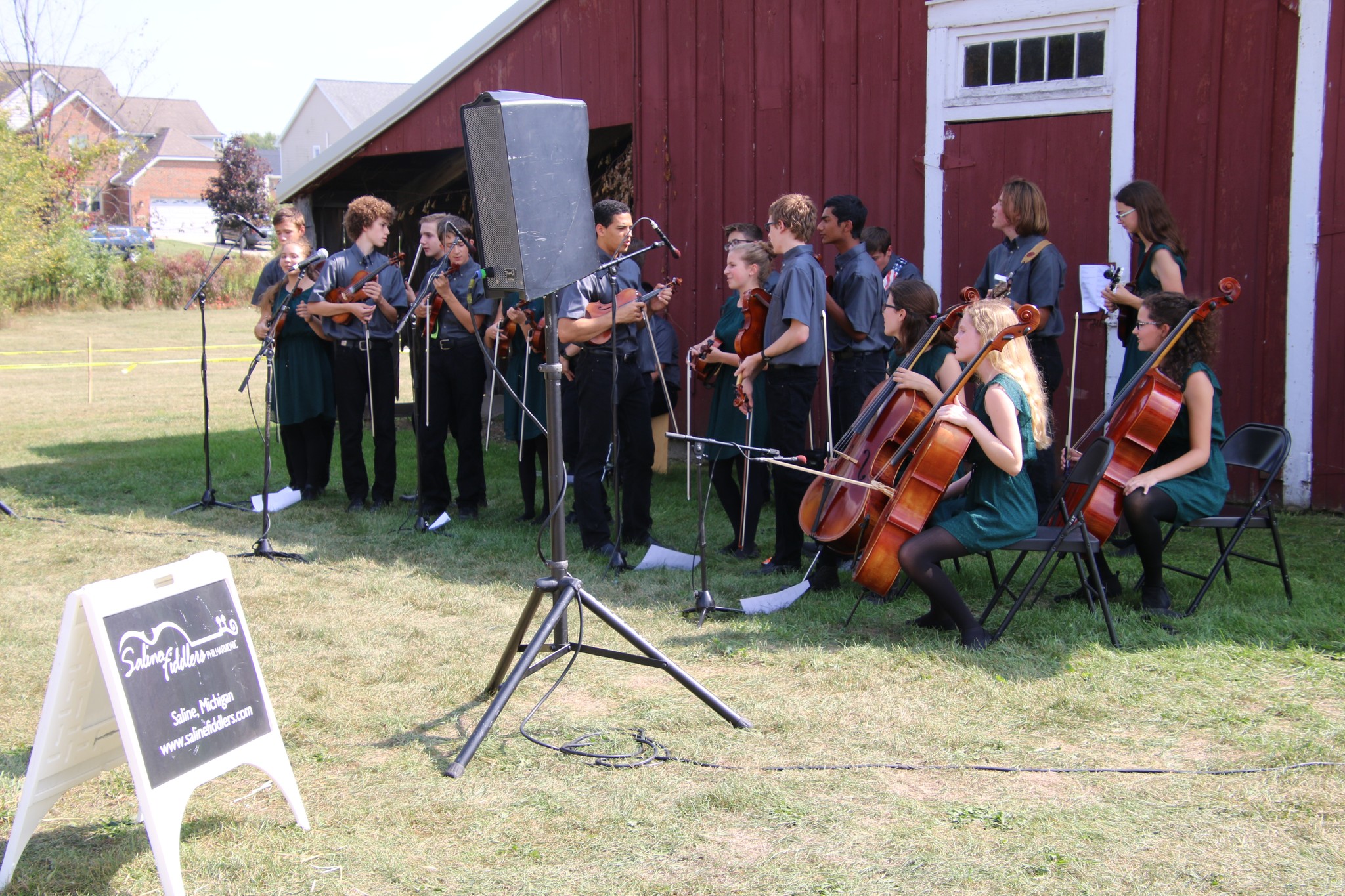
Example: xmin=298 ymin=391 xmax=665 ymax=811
xmin=449 ymin=222 xmax=477 ymax=254
xmin=650 ymin=219 xmax=681 ymax=259
xmin=237 ymin=214 xmax=267 ymax=238
xmin=288 ymin=248 xmax=329 ymax=272
xmin=750 ymin=455 xmax=807 ymax=465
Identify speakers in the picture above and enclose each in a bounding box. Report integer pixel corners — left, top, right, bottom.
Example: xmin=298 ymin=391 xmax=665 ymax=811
xmin=460 ymin=90 xmax=601 ymax=305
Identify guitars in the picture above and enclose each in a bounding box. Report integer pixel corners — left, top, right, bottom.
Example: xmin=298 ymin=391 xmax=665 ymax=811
xmin=1103 ymin=267 xmax=1141 ymax=348
xmin=988 ymin=274 xmax=1015 ymax=300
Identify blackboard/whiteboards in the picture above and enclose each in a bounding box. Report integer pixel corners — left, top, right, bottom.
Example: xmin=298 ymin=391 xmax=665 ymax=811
xmin=21 ymin=549 xmax=273 ymax=790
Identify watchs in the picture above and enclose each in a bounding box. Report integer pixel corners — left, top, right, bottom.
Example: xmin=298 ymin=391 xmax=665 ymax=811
xmin=761 ymin=348 xmax=774 ymax=362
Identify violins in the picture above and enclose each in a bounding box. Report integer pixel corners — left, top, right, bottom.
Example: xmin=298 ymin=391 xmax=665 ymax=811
xmin=256 ymin=285 xmax=302 ymax=359
xmin=326 ymin=251 xmax=405 ymax=328
xmin=582 ymin=277 xmax=683 ymax=346
xmin=421 ymin=264 xmax=459 ymax=339
xmin=492 ymin=294 xmax=532 ymax=357
xmin=730 ymin=287 xmax=775 ymax=408
xmin=522 ymin=307 xmax=547 ymax=362
xmin=693 ymin=338 xmax=723 ymax=389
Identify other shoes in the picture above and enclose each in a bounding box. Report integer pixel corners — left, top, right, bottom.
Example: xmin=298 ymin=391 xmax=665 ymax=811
xmin=904 ymin=611 xmax=957 ymax=632
xmin=369 ymin=500 xmax=390 ymax=514
xmin=419 ymin=499 xmax=577 ymax=525
xmin=961 ymin=628 xmax=992 ymax=656
xmin=776 ymin=573 xmax=842 ymax=594
xmin=400 ymin=492 xmax=419 ymax=503
xmin=1142 ymin=581 xmax=1174 ymax=630
xmin=293 ymin=484 xmax=326 ymax=499
xmin=594 ymin=542 xmax=628 ymax=558
xmin=743 ymin=560 xmax=803 ymax=576
xmin=1054 ymin=570 xmax=1125 ymax=605
xmin=344 ymin=501 xmax=366 ymax=514
xmin=712 ymin=539 xmax=759 ymax=560
xmin=864 ymin=576 xmax=900 ymax=603
xmin=622 ymin=535 xmax=663 ymax=548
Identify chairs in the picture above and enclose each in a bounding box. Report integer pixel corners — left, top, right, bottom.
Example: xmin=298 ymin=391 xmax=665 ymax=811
xmin=978 ymin=436 xmax=1120 ymax=649
xmin=1135 ymin=423 xmax=1292 ymax=616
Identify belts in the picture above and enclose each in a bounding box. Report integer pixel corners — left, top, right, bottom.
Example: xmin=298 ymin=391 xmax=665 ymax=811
xmin=430 ymin=334 xmax=482 ymax=350
xmin=338 ymin=339 xmax=393 ymax=351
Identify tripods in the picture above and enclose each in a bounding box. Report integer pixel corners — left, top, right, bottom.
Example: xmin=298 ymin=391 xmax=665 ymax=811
xmin=446 ymin=296 xmax=749 ymax=778
xmin=233 ymin=269 xmax=308 ymax=560
xmin=662 ymin=431 xmax=781 ymax=628
xmin=174 ymin=225 xmax=255 ymax=511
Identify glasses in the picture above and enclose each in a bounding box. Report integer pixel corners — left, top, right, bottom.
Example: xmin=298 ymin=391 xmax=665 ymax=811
xmin=1116 ymin=209 xmax=1136 ymax=221
xmin=1136 ymin=321 xmax=1171 ymax=330
xmin=765 ymin=221 xmax=789 ymax=232
xmin=882 ymin=302 xmax=901 ymax=313
xmin=724 ymin=239 xmax=754 ymax=251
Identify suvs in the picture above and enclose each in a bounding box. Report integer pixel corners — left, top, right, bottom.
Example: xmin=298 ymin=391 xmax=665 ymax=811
xmin=83 ymin=226 xmax=155 ymax=265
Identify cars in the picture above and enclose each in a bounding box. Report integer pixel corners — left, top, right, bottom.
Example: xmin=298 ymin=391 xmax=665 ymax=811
xmin=216 ymin=213 xmax=277 ymax=249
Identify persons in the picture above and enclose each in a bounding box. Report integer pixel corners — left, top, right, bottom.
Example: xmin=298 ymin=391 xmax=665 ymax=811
xmin=685 ymin=194 xmax=966 ymax=591
xmin=253 ymin=240 xmax=336 ymax=501
xmin=1052 ymin=290 xmax=1231 ymax=622
xmin=896 ymin=299 xmax=1056 ymax=652
xmin=556 ymin=199 xmax=674 ymax=570
xmin=250 ymin=205 xmax=318 ymax=306
xmin=972 ymin=175 xmax=1066 ymax=527
xmin=1100 ymin=179 xmax=1191 ymax=553
xmin=398 ymin=212 xmax=498 ymax=521
xmin=307 ymin=195 xmax=409 ymax=516
xmin=483 ymin=237 xmax=682 ymax=526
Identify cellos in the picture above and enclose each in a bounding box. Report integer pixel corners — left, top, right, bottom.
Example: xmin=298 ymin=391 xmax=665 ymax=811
xmin=805 ymin=252 xmax=834 ymax=456
xmin=799 ymin=284 xmax=980 ymax=582
xmin=844 ymin=303 xmax=1042 ymax=628
xmin=1051 ymin=278 xmax=1245 ymax=551
